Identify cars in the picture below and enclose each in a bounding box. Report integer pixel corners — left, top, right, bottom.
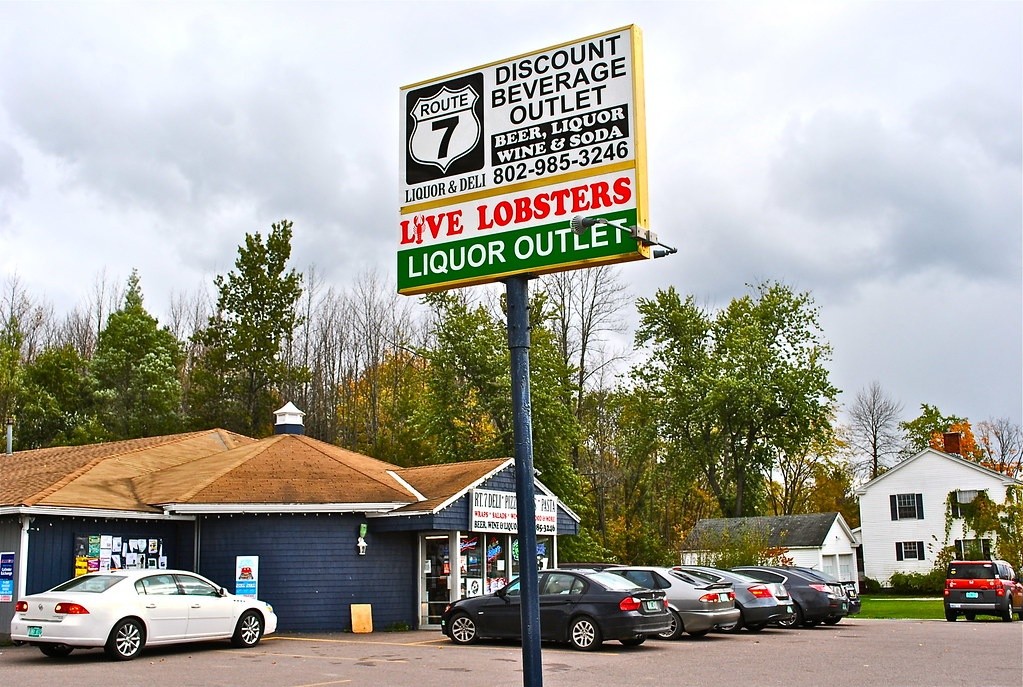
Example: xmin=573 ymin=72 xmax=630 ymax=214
xmin=775 ymin=566 xmax=861 ymax=626
xmin=726 ymin=566 xmax=850 ymax=629
xmin=672 ymin=565 xmax=794 ymax=633
xmin=440 ymin=569 xmax=672 ymax=651
xmin=603 ymin=566 xmax=741 ymax=641
xmin=10 ymin=568 xmax=278 ymax=660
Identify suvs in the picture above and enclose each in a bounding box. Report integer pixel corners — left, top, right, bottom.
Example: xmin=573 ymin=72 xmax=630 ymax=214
xmin=943 ymin=560 xmax=1023 ymax=623
